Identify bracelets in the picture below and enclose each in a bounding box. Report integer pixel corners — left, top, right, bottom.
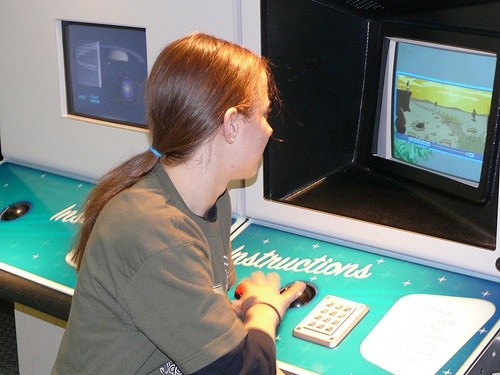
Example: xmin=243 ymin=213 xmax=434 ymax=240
xmin=243 ymin=301 xmax=282 ymax=326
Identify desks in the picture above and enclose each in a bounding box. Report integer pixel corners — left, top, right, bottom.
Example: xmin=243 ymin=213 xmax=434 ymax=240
xmin=229 ymin=219 xmax=500 ymax=375
xmin=0 ymin=159 xmax=248 ymax=375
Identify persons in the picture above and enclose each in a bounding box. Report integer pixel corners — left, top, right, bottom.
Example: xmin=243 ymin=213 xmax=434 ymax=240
xmin=50 ymin=31 xmax=306 ymax=374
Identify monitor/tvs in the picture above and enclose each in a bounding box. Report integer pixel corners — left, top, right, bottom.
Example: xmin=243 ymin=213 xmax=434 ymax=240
xmin=366 ymin=22 xmax=500 ymax=202
xmin=61 ymin=20 xmax=149 ymax=129
